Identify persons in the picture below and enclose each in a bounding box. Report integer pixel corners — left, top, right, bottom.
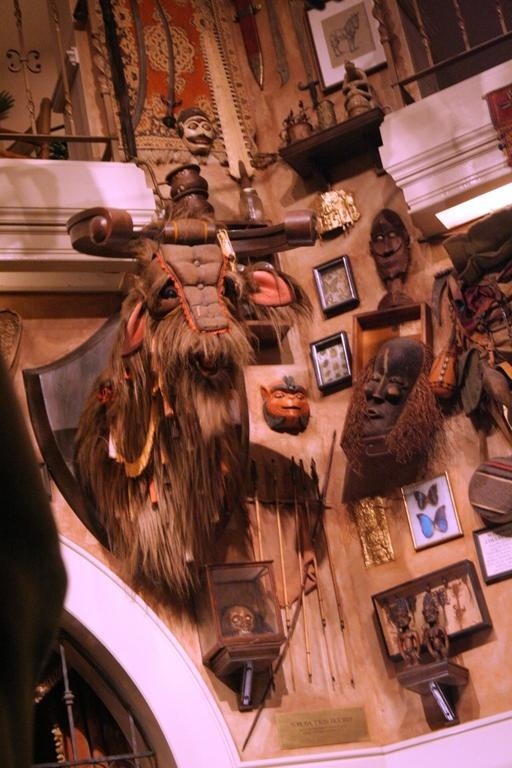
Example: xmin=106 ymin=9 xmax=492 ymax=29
xmin=422 ymin=594 xmax=450 ymax=663
xmin=177 ymin=107 xmax=216 ymax=155
xmin=340 ymin=337 xmax=444 ymax=471
xmin=370 ymin=210 xmax=413 ymax=310
xmin=394 ymin=600 xmax=421 ymax=669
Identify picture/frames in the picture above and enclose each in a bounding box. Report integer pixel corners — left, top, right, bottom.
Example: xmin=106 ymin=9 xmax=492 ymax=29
xmin=472 ymin=520 xmax=512 ymax=584
xmin=304 ymin=0 xmax=388 ymax=95
xmin=310 ymin=330 xmax=353 ymax=393
xmin=399 ymin=469 xmax=464 ymax=553
xmin=313 ymin=253 xmax=360 ymax=315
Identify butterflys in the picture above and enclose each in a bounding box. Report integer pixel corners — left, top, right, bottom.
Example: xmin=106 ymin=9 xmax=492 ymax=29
xmin=413 ymin=483 xmax=438 ymax=510
xmin=416 ymin=504 xmax=448 ymax=539
